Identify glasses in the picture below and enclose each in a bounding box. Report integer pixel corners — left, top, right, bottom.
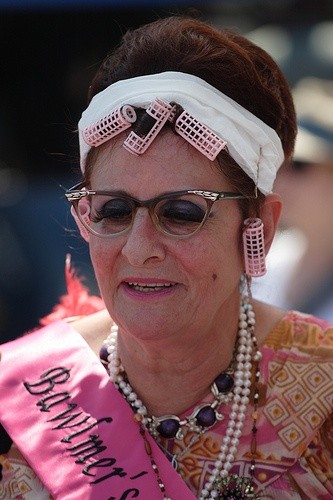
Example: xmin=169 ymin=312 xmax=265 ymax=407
xmin=63 ymin=180 xmax=252 ymax=239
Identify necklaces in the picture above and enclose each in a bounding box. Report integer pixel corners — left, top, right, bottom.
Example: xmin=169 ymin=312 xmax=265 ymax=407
xmin=99 ymin=292 xmax=262 ymax=500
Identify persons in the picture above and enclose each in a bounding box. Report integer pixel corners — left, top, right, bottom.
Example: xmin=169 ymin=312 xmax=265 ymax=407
xmin=243 ymin=75 xmax=333 ymax=325
xmin=1 ymin=16 xmax=333 ymax=500
